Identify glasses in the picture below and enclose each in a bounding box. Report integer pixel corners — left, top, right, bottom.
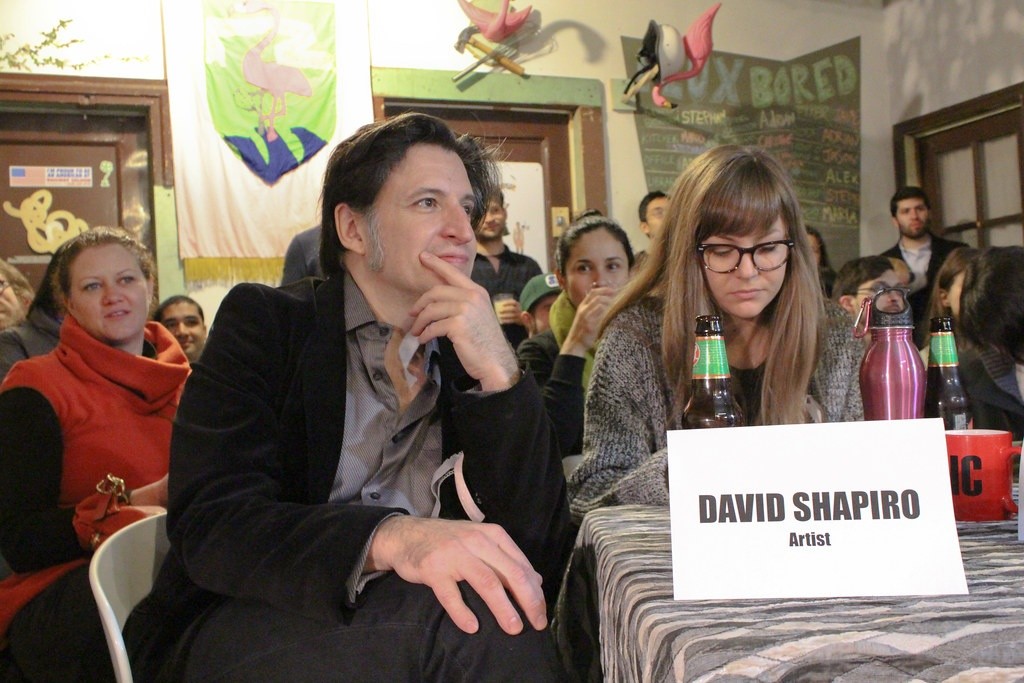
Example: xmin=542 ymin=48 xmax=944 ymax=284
xmin=845 ymin=287 xmax=910 ymax=298
xmin=697 ymin=233 xmax=794 ymax=274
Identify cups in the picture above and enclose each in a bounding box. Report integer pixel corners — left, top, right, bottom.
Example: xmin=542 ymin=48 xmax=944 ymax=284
xmin=584 ymin=281 xmax=618 ymax=294
xmin=492 ymin=293 xmax=514 ymax=325
xmin=943 ymin=428 xmax=1022 ymax=523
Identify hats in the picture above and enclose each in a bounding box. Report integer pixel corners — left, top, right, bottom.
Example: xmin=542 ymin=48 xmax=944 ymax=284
xmin=520 ymin=273 xmax=562 ymax=312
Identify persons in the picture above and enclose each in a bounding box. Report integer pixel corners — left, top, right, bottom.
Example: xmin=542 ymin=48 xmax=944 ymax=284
xmin=801 ymin=187 xmax=1024 ymax=442
xmin=470 ymin=186 xmax=543 ymax=358
xmin=567 ymin=143 xmax=867 ymax=525
xmin=152 ymin=295 xmax=207 ymax=364
xmin=0 ymin=247 xmax=67 ymax=385
xmin=281 ymin=225 xmax=321 ymax=287
xmin=515 ymin=191 xmax=670 ymax=459
xmin=164 ymin=111 xmax=570 ymax=683
xmin=0 ymin=225 xmax=193 ymax=683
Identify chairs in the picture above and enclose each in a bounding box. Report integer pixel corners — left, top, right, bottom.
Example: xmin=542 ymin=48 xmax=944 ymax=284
xmin=90 ymin=512 xmax=171 ymax=683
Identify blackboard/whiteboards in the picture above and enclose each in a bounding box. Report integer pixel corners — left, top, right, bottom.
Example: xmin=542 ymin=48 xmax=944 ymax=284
xmin=621 ymin=36 xmax=862 ymax=272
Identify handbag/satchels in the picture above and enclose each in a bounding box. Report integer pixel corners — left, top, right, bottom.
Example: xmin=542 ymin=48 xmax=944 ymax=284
xmin=72 ymin=472 xmax=168 ymax=550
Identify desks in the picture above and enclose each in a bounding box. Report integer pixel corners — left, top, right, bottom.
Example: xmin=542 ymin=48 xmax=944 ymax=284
xmin=550 ymin=483 xmax=1024 ymax=683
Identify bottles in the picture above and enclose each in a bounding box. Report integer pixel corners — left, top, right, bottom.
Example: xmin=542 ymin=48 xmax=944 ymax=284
xmin=924 ymin=315 xmax=975 ymax=429
xmin=680 ymin=314 xmax=745 ymax=428
xmin=853 ymin=285 xmax=926 ymax=420
